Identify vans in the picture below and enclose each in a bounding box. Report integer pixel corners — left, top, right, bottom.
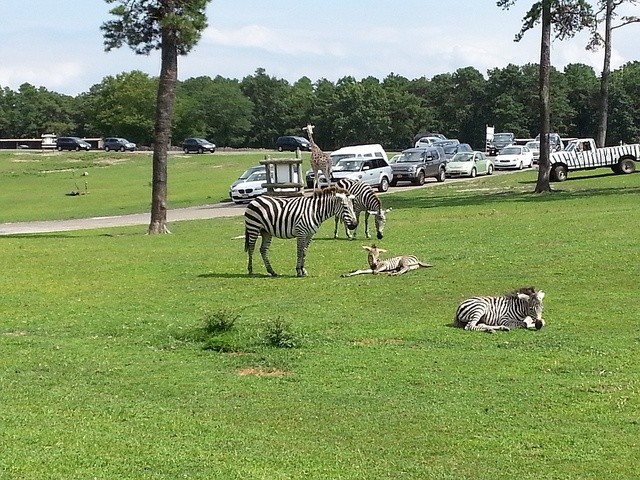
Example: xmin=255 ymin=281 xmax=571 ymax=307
xmin=306 ymin=143 xmax=391 ymax=189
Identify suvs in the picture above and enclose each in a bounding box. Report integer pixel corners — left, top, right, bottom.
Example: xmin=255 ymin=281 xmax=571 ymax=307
xmin=321 ymin=156 xmax=394 ymax=192
xmin=489 ymin=132 xmax=519 ymax=157
xmin=391 ymin=146 xmax=447 ymax=186
xmin=103 ymin=137 xmax=136 ymax=153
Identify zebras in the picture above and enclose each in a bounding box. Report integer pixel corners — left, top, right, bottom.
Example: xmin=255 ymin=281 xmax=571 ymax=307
xmin=244 ymin=185 xmax=359 ymax=278
xmin=456 ymin=287 xmax=545 ymax=333
xmin=340 ymin=243 xmax=434 ymax=279
xmin=331 ymin=177 xmax=390 ymax=240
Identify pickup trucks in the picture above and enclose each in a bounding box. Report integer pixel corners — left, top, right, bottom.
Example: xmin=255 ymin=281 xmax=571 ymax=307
xmin=547 ymin=137 xmax=640 ymax=182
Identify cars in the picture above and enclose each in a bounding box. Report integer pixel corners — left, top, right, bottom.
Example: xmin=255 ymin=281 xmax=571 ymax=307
xmin=414 ymin=136 xmax=442 ymax=147
xmin=535 ymin=132 xmax=579 ymax=161
xmin=525 ymin=140 xmax=555 ymax=163
xmin=428 ymin=139 xmax=460 ymax=152
xmin=56 ymin=136 xmax=92 ymax=151
xmin=445 ymin=151 xmax=494 ymax=178
xmin=230 ymin=170 xmax=305 ymax=205
xmin=440 ymin=143 xmax=473 ymax=161
xmin=492 ymin=145 xmax=534 ymax=170
xmin=181 ymin=137 xmax=216 ymax=154
xmin=229 ymin=164 xmax=298 ymax=195
xmin=275 ymin=135 xmax=311 ymax=152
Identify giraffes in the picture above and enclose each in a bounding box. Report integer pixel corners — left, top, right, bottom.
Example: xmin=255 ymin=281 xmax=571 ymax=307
xmin=302 ymin=123 xmax=333 ymax=194
